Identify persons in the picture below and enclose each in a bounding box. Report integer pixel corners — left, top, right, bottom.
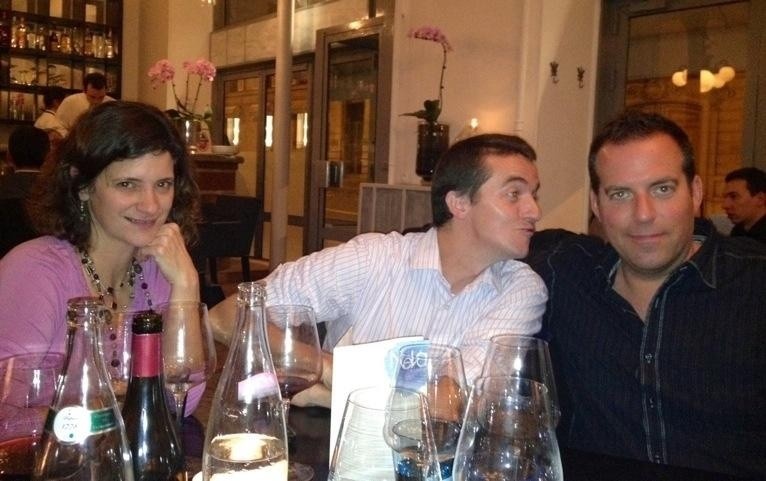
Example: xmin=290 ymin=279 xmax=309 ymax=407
xmin=201 ymin=133 xmax=549 ymax=420
xmin=401 ymin=108 xmax=766 ymax=481
xmin=719 ymin=168 xmax=766 ymax=242
xmin=34 ymin=86 xmax=66 ymax=130
xmin=0 ymin=126 xmax=50 ymax=201
xmin=52 ymin=73 xmax=117 ymax=139
xmin=0 ymin=100 xmax=206 ymax=441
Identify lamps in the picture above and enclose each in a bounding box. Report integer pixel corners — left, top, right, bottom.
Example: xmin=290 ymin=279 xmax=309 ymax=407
xmin=673 ymin=26 xmax=734 ymax=92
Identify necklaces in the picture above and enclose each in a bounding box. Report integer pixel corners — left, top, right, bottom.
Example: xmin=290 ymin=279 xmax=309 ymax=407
xmin=74 ymin=246 xmax=154 ymax=369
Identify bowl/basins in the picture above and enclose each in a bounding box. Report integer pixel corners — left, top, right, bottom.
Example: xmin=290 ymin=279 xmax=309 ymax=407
xmin=212 ymin=145 xmax=233 ymax=154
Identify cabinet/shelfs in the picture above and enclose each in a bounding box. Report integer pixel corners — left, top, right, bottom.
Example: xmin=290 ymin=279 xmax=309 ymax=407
xmin=0 ymin=1 xmax=121 ymax=123
xmin=356 ymin=185 xmax=443 ymax=236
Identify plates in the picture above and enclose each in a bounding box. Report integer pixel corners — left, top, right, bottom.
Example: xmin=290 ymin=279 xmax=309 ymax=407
xmin=193 ymin=461 xmax=315 ymax=481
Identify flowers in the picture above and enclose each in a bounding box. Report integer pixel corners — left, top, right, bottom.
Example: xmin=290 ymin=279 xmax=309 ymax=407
xmin=148 ymin=59 xmax=214 ymax=116
xmin=397 ymin=27 xmax=451 ymax=125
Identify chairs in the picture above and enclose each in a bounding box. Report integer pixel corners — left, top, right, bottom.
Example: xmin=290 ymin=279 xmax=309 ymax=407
xmin=195 ymin=193 xmax=258 ymax=284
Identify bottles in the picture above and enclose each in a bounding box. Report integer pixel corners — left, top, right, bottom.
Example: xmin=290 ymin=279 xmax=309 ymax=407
xmin=33 ymin=296 xmax=134 ymax=481
xmin=105 ymin=310 xmax=187 ymax=481
xmin=203 ymin=280 xmax=290 ymax=481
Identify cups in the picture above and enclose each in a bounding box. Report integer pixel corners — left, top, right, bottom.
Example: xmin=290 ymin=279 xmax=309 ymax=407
xmin=161 ymin=302 xmax=217 ymax=469
xmin=475 ymin=334 xmax=559 ymax=476
xmin=328 ymin=387 xmax=442 ymax=481
xmin=451 ymin=376 xmax=564 ymax=481
xmin=261 ymin=306 xmax=323 ymax=440
xmin=0 ymin=352 xmax=87 ymax=481
xmin=383 ymin=345 xmax=475 ymax=479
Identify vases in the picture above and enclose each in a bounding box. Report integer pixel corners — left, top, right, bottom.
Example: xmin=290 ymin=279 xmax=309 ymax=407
xmin=172 ymin=117 xmax=213 ymax=154
xmin=416 ymin=123 xmax=450 ymax=186
